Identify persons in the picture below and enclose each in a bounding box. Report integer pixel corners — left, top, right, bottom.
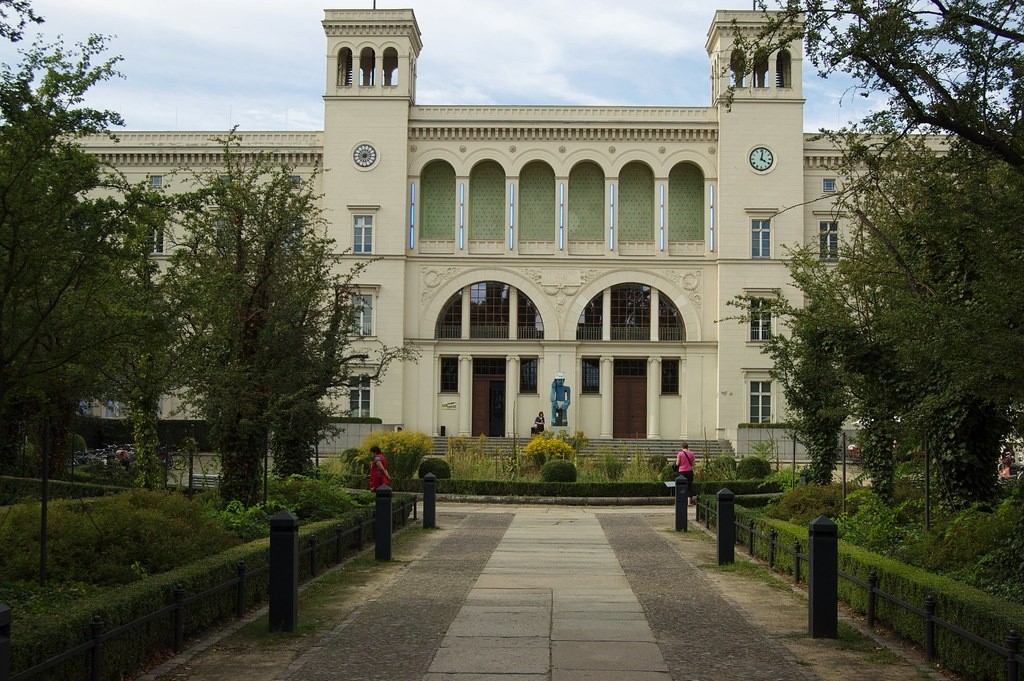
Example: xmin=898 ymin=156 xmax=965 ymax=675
xmin=368 ymin=445 xmax=392 ymax=490
xmin=550 ymin=372 xmax=570 ymax=422
xmin=534 ymin=412 xmax=545 ymax=436
xmin=675 ymin=443 xmax=696 ymax=507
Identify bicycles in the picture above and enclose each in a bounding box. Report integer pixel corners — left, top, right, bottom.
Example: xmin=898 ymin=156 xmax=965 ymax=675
xmin=64 ymin=441 xmax=188 ymax=473
